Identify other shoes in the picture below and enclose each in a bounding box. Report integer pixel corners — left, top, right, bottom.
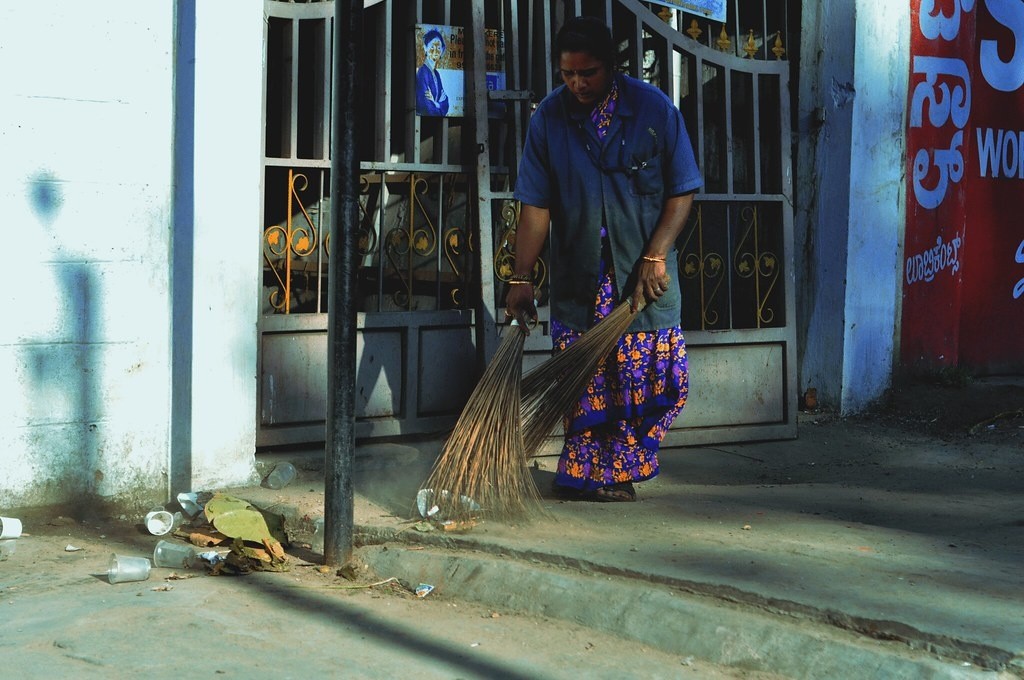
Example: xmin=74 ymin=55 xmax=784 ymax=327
xmin=558 ymin=478 xmax=637 ymax=503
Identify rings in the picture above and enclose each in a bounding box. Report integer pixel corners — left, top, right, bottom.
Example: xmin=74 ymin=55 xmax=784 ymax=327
xmin=505 ymin=311 xmax=514 ymax=317
xmin=654 ymin=287 xmax=661 ymax=292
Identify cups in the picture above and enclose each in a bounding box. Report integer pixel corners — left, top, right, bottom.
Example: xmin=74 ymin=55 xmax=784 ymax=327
xmin=0 ymin=517 xmax=22 ymax=540
xmin=0 ymin=541 xmax=17 ymax=559
xmin=107 ymin=553 xmax=150 ymax=585
xmin=153 ymin=540 xmax=195 ymax=570
xmin=266 ymin=460 xmax=298 ymax=490
xmin=143 ymin=510 xmax=174 ymax=536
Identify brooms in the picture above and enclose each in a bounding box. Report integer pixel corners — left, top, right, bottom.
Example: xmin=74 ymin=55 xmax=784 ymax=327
xmin=410 ymin=283 xmax=561 ymax=527
xmin=518 ymin=272 xmax=673 ymax=466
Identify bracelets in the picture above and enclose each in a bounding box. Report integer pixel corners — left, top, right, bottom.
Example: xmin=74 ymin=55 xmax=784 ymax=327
xmin=642 ymin=255 xmax=666 ymax=262
xmin=508 ymin=274 xmax=535 ymax=285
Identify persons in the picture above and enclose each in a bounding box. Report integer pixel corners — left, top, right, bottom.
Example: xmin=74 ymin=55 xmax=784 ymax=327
xmin=504 ymin=16 xmax=704 ymax=503
xmin=416 ymin=28 xmax=449 ymax=117
